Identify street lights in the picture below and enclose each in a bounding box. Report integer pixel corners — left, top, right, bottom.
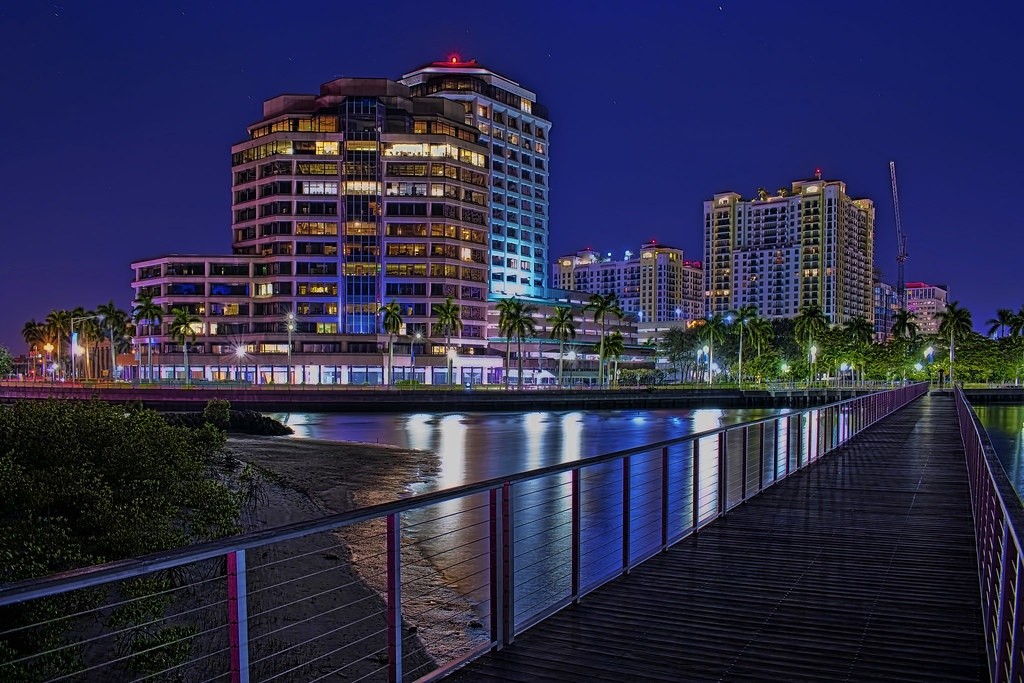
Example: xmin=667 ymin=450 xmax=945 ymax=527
xmin=927 ymin=346 xmax=935 ymax=387
xmin=447 ymin=350 xmax=454 ymax=384
xmin=569 ymin=350 xmax=575 ymax=390
xmin=709 ymin=317 xmax=731 ymax=385
xmin=738 ymin=318 xmax=748 ymax=384
xmin=123 ymin=334 xmax=139 ymax=380
xmin=71 ymin=315 xmax=104 ymax=382
xmin=237 ymin=346 xmax=245 ymax=383
xmin=286 ymin=313 xmax=295 ymax=384
xmin=410 ymin=333 xmax=421 ymax=386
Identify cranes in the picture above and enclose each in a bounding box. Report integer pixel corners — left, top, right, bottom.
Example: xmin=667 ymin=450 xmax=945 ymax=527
xmin=888 ymin=160 xmax=910 ymax=315
